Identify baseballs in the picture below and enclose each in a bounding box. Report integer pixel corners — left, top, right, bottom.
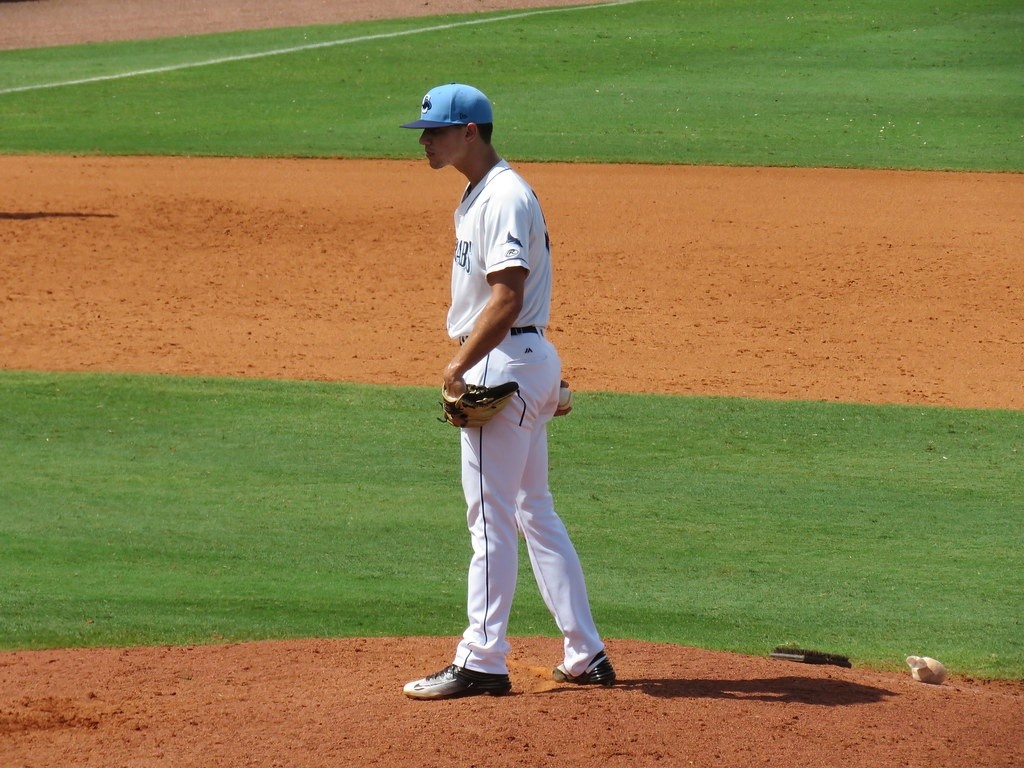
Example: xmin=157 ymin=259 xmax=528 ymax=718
xmin=557 ymin=387 xmax=572 ymax=410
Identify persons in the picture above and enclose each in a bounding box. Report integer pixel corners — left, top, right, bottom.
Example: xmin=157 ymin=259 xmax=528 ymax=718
xmin=399 ymin=82 xmax=617 ymax=698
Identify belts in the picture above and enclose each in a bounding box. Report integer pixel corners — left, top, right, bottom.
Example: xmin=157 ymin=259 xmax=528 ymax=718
xmin=459 ymin=327 xmax=543 ymax=345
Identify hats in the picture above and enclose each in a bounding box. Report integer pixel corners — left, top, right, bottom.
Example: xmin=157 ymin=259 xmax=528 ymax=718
xmin=398 ymin=82 xmax=492 ymax=128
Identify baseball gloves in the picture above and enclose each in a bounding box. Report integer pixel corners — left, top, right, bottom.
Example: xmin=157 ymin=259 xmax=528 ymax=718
xmin=436 ymin=381 xmax=527 ymax=428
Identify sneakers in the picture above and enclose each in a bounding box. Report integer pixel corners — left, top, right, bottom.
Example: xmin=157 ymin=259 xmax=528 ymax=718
xmin=403 ymin=663 xmax=512 ymax=699
xmin=552 ymin=650 xmax=616 ymax=684
